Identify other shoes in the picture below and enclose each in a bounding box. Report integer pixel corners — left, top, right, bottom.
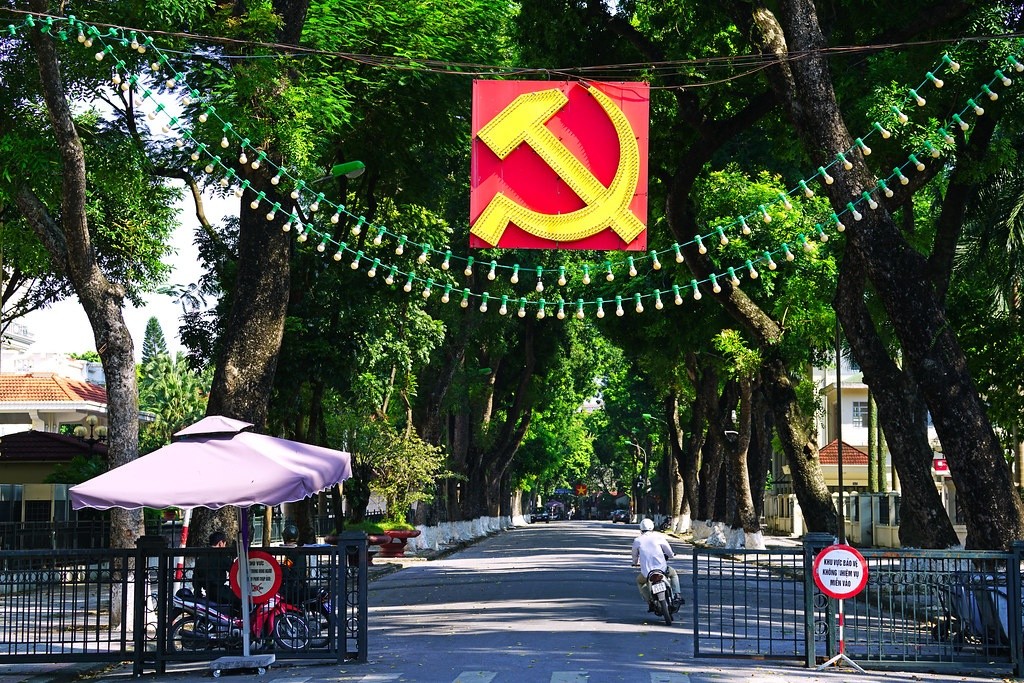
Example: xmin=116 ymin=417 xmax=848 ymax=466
xmin=674 ymin=594 xmax=685 ymax=604
xmin=648 ymin=602 xmax=655 ymax=612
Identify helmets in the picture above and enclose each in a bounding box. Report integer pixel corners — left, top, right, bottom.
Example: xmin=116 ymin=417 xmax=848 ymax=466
xmin=281 ymin=525 xmax=299 ymax=542
xmin=640 ymin=518 xmax=654 ymax=531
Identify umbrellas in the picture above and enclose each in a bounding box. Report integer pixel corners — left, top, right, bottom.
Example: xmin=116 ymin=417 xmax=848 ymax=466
xmin=69 ymin=416 xmax=353 ymax=656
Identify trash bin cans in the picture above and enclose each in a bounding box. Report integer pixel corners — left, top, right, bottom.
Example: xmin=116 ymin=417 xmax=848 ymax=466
xmin=798 ymin=532 xmax=835 ymax=554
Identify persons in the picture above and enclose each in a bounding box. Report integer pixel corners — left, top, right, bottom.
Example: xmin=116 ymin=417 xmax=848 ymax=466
xmin=568 ymin=504 xmax=575 ymax=520
xmin=192 ymin=533 xmax=237 ymax=651
xmin=259 ymin=525 xmax=306 ymax=650
xmin=632 ymin=519 xmax=683 ymax=613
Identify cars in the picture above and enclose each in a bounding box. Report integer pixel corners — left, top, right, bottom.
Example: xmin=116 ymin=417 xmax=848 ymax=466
xmin=530 ymin=507 xmax=550 ymax=523
xmin=557 ymin=510 xmax=576 ymax=520
xmin=612 ymin=509 xmax=631 ymax=525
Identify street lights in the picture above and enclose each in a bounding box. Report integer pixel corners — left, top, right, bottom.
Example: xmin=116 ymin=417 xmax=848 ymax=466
xmin=625 ymin=440 xmax=648 ymax=522
xmin=642 ymin=413 xmax=674 ymax=517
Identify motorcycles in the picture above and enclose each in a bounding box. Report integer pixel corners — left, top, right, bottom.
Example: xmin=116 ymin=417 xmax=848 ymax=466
xmin=631 ymin=552 xmax=682 ymax=626
xmin=170 ymin=581 xmax=313 ymax=655
xmin=286 ymin=585 xmax=331 ymax=649
